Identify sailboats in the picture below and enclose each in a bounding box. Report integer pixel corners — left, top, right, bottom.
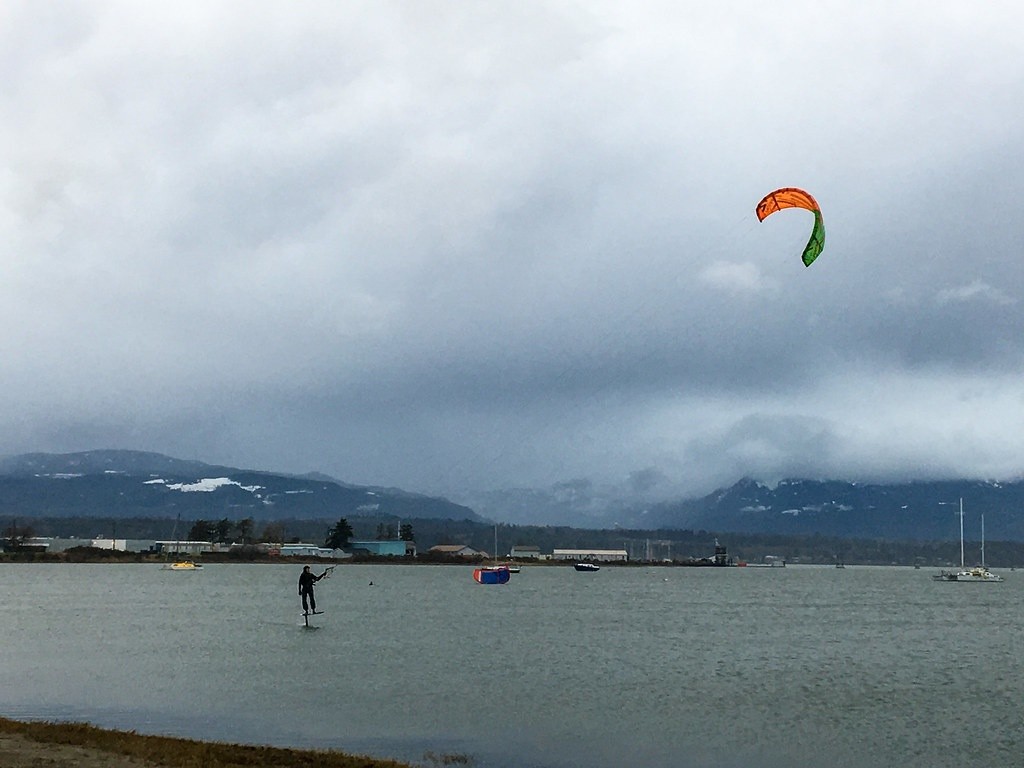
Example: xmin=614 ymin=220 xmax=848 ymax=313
xmin=931 ymin=496 xmax=1005 ymax=583
xmin=480 ymin=524 xmax=521 ymax=574
xmin=159 ymin=512 xmax=205 ymax=571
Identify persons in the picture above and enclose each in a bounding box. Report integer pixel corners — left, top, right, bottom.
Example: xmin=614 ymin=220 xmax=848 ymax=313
xmin=298 ymin=565 xmax=323 ymax=616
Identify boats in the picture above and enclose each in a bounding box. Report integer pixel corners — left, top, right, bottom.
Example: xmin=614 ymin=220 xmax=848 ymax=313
xmin=573 ymin=563 xmax=600 ymax=571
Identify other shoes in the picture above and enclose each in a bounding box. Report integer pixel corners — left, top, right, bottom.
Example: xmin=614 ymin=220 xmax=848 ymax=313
xmin=306 ymin=610 xmax=308 ymax=614
xmin=312 ymin=609 xmax=316 ymax=614
